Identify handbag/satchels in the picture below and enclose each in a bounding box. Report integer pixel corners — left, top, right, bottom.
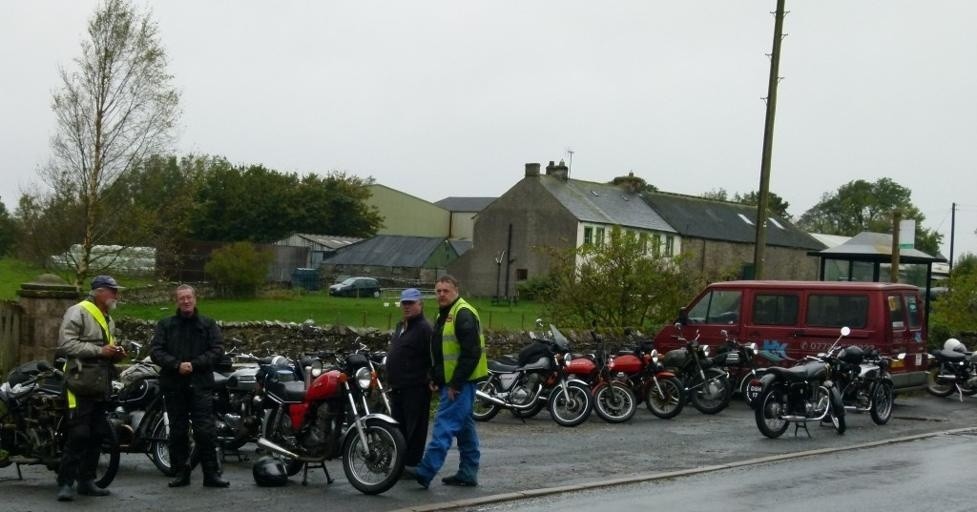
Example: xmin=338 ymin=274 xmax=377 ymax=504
xmin=63 ymin=359 xmax=113 ymax=398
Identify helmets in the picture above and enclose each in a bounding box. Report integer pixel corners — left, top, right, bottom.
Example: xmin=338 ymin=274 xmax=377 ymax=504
xmin=253 ymin=454 xmax=288 ymax=486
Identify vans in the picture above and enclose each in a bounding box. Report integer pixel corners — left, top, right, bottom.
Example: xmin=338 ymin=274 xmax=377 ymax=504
xmin=645 ymin=278 xmax=930 ymax=399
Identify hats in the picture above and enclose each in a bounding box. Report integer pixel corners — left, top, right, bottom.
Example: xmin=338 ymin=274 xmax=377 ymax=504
xmin=91 ymin=274 xmax=126 ymax=290
xmin=400 ymin=287 xmax=423 ymax=302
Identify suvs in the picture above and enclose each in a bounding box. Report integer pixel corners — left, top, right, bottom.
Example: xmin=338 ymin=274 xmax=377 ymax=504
xmin=328 ymin=277 xmax=382 ymax=299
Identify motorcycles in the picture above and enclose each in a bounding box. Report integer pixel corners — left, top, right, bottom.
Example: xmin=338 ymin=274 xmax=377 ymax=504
xmin=944 ymin=339 xmax=977 ymax=396
xmin=0 ymin=317 xmax=407 ymax=495
xmin=511 ymin=317 xmax=758 ymax=427
xmin=468 ymin=317 xmax=593 ymax=427
xmin=924 ymin=338 xmax=977 ymax=404
xmin=808 ymin=342 xmax=895 ymax=424
xmin=748 ymin=325 xmax=853 ymax=439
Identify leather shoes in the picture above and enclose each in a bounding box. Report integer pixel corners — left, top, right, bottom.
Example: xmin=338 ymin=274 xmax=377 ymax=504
xmin=443 ymin=474 xmax=478 ymax=485
xmin=404 ymin=464 xmax=429 ymax=488
xmin=203 ymin=472 xmax=230 ymax=487
xmin=78 ymin=483 xmax=111 ymax=496
xmin=57 ymin=484 xmax=74 ymax=500
xmin=169 ymin=472 xmax=191 ymax=488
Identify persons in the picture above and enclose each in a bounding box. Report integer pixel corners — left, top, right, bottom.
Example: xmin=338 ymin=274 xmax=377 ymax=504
xmin=385 ymin=288 xmax=431 ymax=468
xmin=149 ymin=284 xmax=232 ymax=488
xmin=405 ymin=275 xmax=489 ymax=489
xmin=56 ymin=275 xmax=127 ymax=502
